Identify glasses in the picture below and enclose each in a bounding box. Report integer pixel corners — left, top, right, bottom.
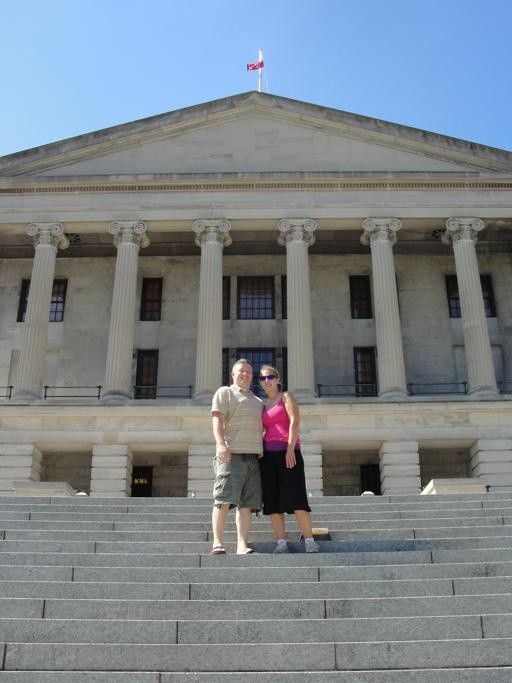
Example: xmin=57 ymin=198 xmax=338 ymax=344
xmin=259 ymin=375 xmax=278 ymax=381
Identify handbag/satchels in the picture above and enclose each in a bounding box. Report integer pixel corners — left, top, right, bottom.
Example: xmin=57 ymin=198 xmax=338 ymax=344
xmin=303 ymin=527 xmax=331 ymax=541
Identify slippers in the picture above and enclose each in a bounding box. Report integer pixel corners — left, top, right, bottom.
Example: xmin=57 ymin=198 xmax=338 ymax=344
xmin=211 ymin=546 xmax=225 ymax=554
xmin=246 ymin=549 xmax=256 ymax=554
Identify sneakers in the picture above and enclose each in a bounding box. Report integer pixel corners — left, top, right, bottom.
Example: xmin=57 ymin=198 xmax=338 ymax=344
xmin=305 ymin=541 xmax=321 ymax=552
xmin=273 ymin=544 xmax=290 ymax=553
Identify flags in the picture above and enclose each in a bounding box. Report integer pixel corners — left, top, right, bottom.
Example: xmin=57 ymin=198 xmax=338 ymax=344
xmin=246 ymin=49 xmax=265 ymax=72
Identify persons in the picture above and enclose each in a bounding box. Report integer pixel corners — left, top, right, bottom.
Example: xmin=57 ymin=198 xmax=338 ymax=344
xmin=209 ymin=357 xmax=266 ymax=556
xmin=258 ymin=362 xmax=322 ymax=554
